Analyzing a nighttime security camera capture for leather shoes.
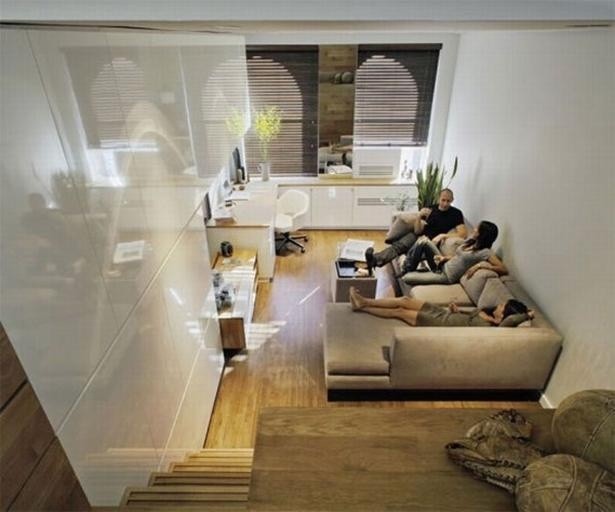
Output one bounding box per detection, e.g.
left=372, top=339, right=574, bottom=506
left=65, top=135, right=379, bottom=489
left=365, top=247, right=376, bottom=268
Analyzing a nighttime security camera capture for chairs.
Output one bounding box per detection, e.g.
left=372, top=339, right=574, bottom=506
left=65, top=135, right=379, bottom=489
left=275, top=189, right=310, bottom=255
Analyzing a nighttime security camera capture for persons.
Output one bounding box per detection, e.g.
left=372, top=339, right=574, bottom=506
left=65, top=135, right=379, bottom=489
left=21, top=193, right=66, bottom=238
left=366, top=189, right=508, bottom=285
left=349, top=286, right=535, bottom=327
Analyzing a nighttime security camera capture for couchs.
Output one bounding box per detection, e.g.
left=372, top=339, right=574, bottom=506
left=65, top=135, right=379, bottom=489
left=320, top=209, right=565, bottom=397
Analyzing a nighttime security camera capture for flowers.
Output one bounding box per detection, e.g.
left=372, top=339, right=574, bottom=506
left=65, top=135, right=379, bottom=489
left=253, top=104, right=283, bottom=162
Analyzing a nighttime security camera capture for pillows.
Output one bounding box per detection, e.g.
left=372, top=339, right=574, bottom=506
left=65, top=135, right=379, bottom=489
left=384, top=215, right=417, bottom=245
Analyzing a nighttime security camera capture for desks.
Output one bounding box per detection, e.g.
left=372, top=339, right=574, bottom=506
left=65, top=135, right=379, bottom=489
left=208, top=180, right=280, bottom=285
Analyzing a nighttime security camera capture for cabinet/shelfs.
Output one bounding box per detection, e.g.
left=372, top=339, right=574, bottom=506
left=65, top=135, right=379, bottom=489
left=210, top=248, right=261, bottom=353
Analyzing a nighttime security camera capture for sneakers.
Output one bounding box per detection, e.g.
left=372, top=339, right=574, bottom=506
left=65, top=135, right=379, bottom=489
left=350, top=286, right=369, bottom=312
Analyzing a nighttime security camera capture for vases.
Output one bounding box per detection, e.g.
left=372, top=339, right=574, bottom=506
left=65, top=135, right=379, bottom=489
left=259, top=163, right=270, bottom=181
